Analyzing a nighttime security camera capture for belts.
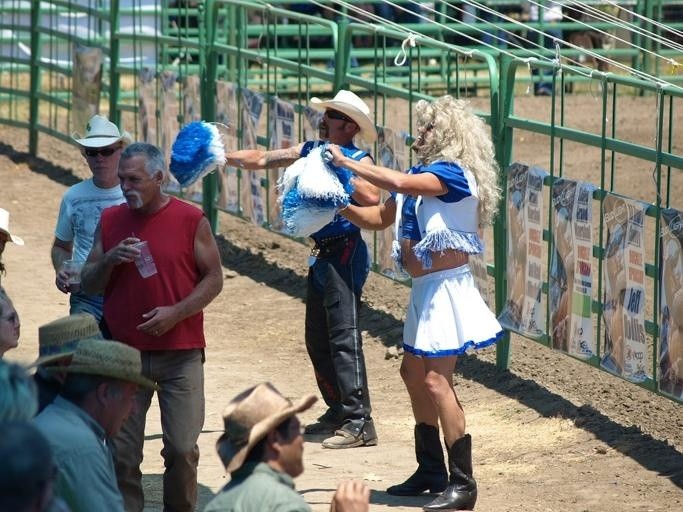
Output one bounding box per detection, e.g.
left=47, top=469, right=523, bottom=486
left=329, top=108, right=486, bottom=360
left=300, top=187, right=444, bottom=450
left=307, top=231, right=361, bottom=268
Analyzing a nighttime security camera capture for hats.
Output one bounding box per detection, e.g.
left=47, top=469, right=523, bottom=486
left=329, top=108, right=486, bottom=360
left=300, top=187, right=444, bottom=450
left=0, top=208, right=24, bottom=246
left=44, top=337, right=161, bottom=391
left=214, top=381, right=319, bottom=474
left=70, top=115, right=131, bottom=151
left=309, top=89, right=378, bottom=144
left=25, top=312, right=104, bottom=370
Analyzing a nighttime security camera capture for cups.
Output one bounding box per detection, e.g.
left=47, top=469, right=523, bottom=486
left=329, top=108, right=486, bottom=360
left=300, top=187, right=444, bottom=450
left=62, top=259, right=82, bottom=294
left=129, top=241, right=157, bottom=280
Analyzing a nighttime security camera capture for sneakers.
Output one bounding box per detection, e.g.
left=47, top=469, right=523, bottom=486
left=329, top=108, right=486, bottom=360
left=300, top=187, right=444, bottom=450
left=303, top=422, right=341, bottom=434
left=321, top=428, right=378, bottom=449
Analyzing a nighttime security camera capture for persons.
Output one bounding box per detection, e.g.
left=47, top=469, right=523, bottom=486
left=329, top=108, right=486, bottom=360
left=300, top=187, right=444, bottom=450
left=0, top=414, right=67, bottom=511
left=80, top=144, right=224, bottom=512
left=0, top=285, right=20, bottom=365
left=49, top=116, right=134, bottom=323
left=521, top=0, right=567, bottom=97
left=324, top=97, right=501, bottom=511
left=30, top=338, right=142, bottom=512
left=202, top=381, right=370, bottom=511
left=223, top=90, right=379, bottom=449
left=0, top=227, right=8, bottom=291
left=580, top=2, right=623, bottom=89
left=0, top=356, right=35, bottom=428
left=27, top=313, right=108, bottom=420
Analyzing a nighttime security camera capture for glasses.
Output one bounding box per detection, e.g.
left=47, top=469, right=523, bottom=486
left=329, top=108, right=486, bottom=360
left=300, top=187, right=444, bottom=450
left=83, top=146, right=121, bottom=157
left=323, top=110, right=352, bottom=123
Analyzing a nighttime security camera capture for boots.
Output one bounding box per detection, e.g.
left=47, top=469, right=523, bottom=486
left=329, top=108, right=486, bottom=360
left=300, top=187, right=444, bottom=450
left=385, top=422, right=448, bottom=496
left=422, top=433, right=477, bottom=511
